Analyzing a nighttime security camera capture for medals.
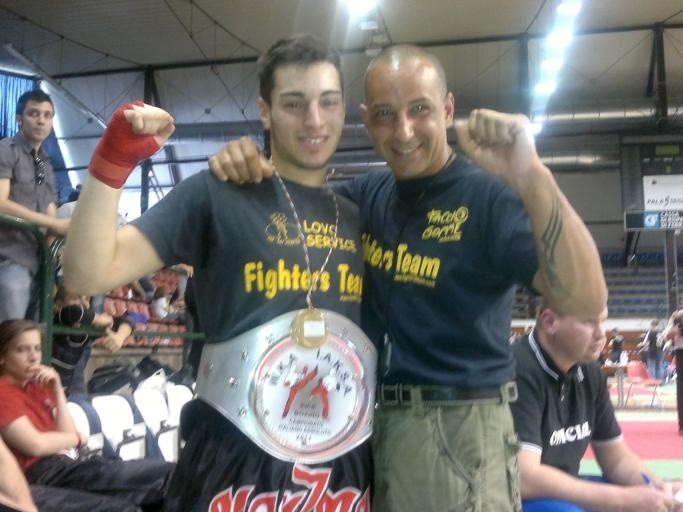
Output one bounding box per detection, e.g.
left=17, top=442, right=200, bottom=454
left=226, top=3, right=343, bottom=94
left=288, top=308, right=330, bottom=350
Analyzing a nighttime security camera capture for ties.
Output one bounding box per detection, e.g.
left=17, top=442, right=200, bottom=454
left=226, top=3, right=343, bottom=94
left=28, top=153, right=52, bottom=186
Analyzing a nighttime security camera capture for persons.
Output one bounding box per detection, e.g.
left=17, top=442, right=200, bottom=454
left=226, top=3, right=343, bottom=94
left=208, top=43, right=608, bottom=512
left=509, top=308, right=683, bottom=436
left=1, top=183, right=203, bottom=512
left=508, top=294, right=683, bottom=512
left=63, top=34, right=388, bottom=512
left=0, top=90, right=71, bottom=321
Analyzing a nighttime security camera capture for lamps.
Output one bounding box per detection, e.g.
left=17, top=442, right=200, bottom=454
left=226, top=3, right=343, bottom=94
left=365, top=43, right=383, bottom=56
left=360, top=14, right=379, bottom=30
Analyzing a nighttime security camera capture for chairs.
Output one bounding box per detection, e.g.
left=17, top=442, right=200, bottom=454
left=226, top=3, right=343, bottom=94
left=94, top=395, right=154, bottom=461
left=102, top=270, right=188, bottom=347
left=605, top=266, right=683, bottom=316
left=166, top=386, right=195, bottom=411
left=624, top=361, right=662, bottom=407
left=135, top=388, right=178, bottom=464
left=62, top=400, right=105, bottom=462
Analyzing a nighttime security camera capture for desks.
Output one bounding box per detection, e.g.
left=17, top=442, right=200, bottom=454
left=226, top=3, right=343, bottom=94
left=603, top=365, right=627, bottom=409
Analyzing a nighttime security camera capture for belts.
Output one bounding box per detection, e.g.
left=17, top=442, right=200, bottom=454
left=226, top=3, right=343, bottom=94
left=372, top=382, right=519, bottom=403
